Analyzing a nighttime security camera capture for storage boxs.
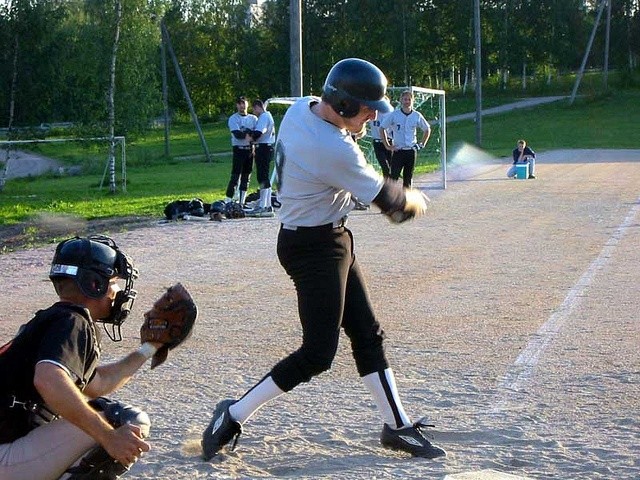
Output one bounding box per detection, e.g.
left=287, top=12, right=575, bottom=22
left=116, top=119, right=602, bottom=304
left=516, top=162, right=530, bottom=179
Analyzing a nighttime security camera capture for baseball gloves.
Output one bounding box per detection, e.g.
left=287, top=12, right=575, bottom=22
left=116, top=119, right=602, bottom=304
left=141, top=281, right=197, bottom=370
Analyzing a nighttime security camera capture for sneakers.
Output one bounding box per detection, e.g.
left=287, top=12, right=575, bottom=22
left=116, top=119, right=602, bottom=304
left=239, top=203, right=253, bottom=211
left=255, top=207, right=275, bottom=217
left=380, top=414, right=447, bottom=461
left=528, top=175, right=537, bottom=179
left=200, top=397, right=243, bottom=461
left=244, top=206, right=263, bottom=217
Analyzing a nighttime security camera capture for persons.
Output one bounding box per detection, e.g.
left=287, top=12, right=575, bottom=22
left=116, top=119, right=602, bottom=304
left=507, top=139, right=537, bottom=179
left=369, top=95, right=397, bottom=180
left=251, top=100, right=278, bottom=212
left=380, top=91, right=432, bottom=193
left=201, top=57, right=448, bottom=463
left=224, top=95, right=258, bottom=211
left=0, top=233, right=164, bottom=480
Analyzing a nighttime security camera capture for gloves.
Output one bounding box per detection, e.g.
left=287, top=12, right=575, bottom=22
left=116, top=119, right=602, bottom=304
left=413, top=142, right=424, bottom=152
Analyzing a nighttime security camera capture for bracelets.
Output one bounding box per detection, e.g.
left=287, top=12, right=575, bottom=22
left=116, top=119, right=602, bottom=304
left=135, top=338, right=160, bottom=363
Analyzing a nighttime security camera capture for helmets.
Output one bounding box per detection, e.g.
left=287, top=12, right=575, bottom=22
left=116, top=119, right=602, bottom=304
left=323, top=58, right=394, bottom=116
left=48, top=234, right=139, bottom=343
left=186, top=197, right=204, bottom=217
left=225, top=201, right=245, bottom=219
left=209, top=200, right=226, bottom=221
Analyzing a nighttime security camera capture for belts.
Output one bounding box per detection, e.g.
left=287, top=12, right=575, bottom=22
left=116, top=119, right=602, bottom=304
left=280, top=214, right=349, bottom=236
left=373, top=139, right=391, bottom=143
